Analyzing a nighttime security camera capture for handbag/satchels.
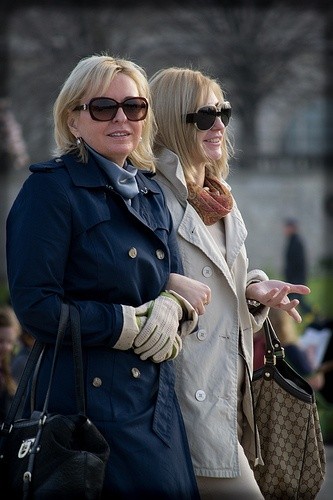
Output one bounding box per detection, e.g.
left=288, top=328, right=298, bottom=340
left=252, top=318, right=326, bottom=500
left=0, top=304, right=109, bottom=499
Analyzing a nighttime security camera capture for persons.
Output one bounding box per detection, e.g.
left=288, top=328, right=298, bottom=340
left=0, top=306, right=34, bottom=419
left=149, top=67, right=312, bottom=500
left=269, top=307, right=324, bottom=389
left=283, top=218, right=313, bottom=314
left=6, top=56, right=199, bottom=500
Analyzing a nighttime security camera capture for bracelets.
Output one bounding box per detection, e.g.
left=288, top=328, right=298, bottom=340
left=247, top=300, right=260, bottom=307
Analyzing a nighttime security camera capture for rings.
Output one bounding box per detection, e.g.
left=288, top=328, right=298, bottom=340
left=204, top=301, right=207, bottom=304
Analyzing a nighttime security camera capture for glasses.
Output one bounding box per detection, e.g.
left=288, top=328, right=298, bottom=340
left=71, top=96, right=148, bottom=123
left=179, top=101, right=231, bottom=131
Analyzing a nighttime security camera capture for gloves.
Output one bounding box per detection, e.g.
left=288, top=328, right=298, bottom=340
left=134, top=294, right=186, bottom=361
left=133, top=315, right=182, bottom=361
